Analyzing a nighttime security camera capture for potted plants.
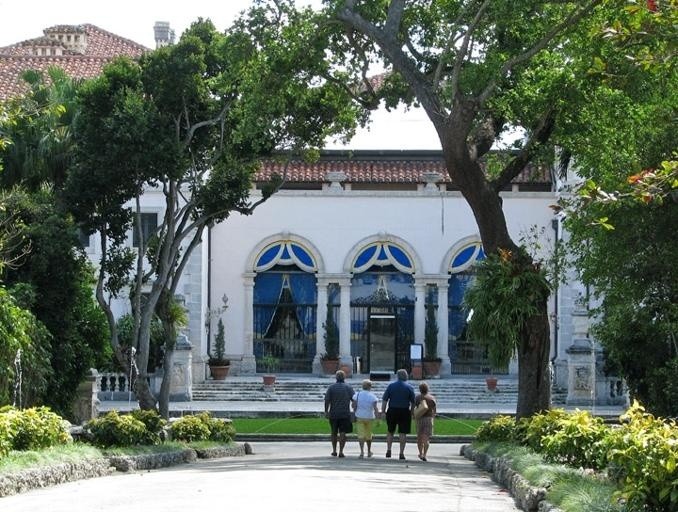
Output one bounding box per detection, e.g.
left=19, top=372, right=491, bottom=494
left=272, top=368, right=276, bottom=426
left=207, top=317, right=231, bottom=379
left=257, top=353, right=281, bottom=386
left=421, top=285, right=442, bottom=377
left=319, top=303, right=341, bottom=374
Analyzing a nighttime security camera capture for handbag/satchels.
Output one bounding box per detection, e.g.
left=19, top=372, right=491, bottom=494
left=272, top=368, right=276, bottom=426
left=350, top=412, right=356, bottom=423
left=414, top=399, right=429, bottom=420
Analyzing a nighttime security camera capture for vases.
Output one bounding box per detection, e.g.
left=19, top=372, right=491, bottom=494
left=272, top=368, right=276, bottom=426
left=486, top=378, right=498, bottom=390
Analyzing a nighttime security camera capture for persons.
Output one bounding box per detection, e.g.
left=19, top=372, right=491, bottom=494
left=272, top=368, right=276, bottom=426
left=381, top=367, right=415, bottom=458
left=323, top=370, right=355, bottom=457
left=410, top=382, right=437, bottom=462
left=352, top=378, right=380, bottom=457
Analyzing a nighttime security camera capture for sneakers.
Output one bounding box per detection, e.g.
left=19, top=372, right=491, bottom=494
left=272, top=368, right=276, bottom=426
left=332, top=452, right=337, bottom=456
left=400, top=454, right=405, bottom=459
left=360, top=451, right=374, bottom=457
left=339, top=453, right=344, bottom=457
left=418, top=454, right=427, bottom=461
left=386, top=452, right=391, bottom=458
